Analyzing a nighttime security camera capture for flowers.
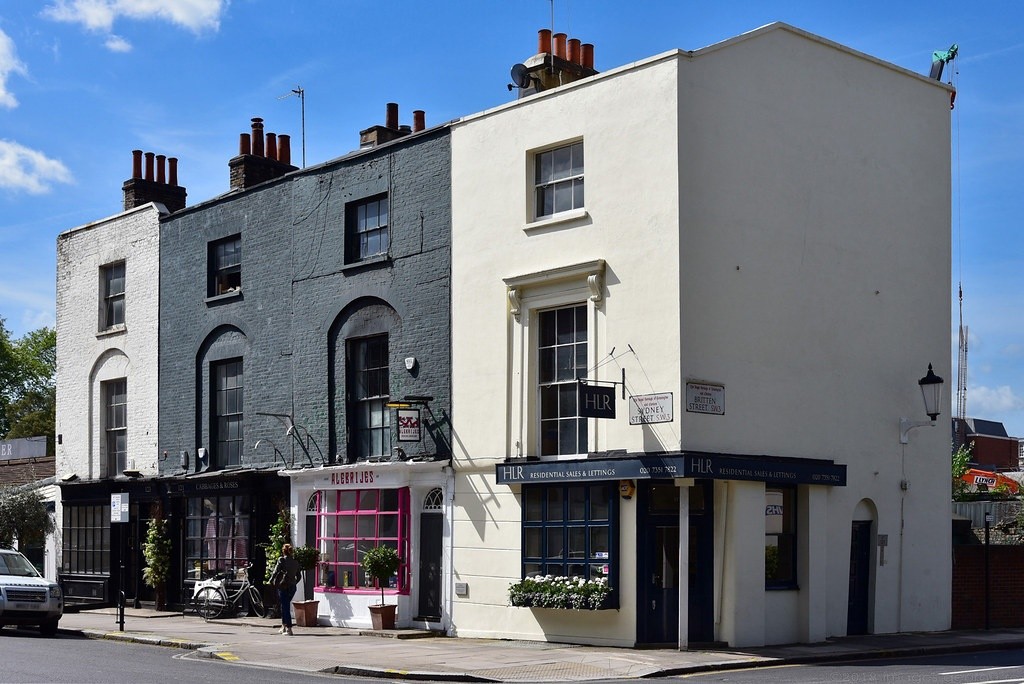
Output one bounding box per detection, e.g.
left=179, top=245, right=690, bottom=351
left=507, top=575, right=612, bottom=611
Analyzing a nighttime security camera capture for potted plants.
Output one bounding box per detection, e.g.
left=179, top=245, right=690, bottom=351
left=292, top=544, right=320, bottom=627
left=362, top=545, right=400, bottom=630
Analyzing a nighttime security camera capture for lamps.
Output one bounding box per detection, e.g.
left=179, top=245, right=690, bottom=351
left=899, top=363, right=944, bottom=445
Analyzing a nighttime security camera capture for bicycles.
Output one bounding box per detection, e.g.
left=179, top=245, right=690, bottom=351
left=194, top=563, right=266, bottom=619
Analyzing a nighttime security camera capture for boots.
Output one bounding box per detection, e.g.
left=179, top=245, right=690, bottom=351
left=282, top=626, right=293, bottom=636
left=278, top=623, right=287, bottom=634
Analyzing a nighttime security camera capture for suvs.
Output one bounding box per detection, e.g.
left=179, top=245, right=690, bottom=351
left=0, top=542, right=64, bottom=638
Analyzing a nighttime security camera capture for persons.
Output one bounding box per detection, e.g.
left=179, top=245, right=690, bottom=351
left=268, top=544, right=301, bottom=635
left=203, top=497, right=247, bottom=570
